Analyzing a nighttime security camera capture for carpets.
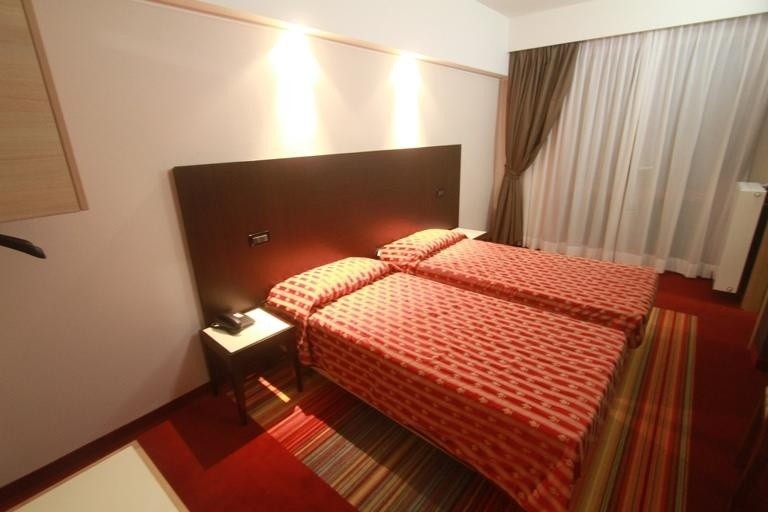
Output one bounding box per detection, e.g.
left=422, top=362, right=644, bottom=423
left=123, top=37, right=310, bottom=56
left=224, top=305, right=699, bottom=512
left=4, top=440, right=191, bottom=512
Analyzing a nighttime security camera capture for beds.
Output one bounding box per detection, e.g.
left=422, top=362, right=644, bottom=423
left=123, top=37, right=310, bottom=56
left=264, top=254, right=630, bottom=511
left=377, top=229, right=659, bottom=347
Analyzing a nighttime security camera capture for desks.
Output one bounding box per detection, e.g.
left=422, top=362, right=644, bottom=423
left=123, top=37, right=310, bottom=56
left=199, top=305, right=303, bottom=426
left=451, top=227, right=489, bottom=243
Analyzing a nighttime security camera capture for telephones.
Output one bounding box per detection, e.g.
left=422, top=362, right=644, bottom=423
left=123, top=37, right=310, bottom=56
left=208, top=310, right=256, bottom=335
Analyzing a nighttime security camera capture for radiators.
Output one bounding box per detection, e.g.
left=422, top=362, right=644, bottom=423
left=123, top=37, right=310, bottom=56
left=709, top=181, right=768, bottom=300
